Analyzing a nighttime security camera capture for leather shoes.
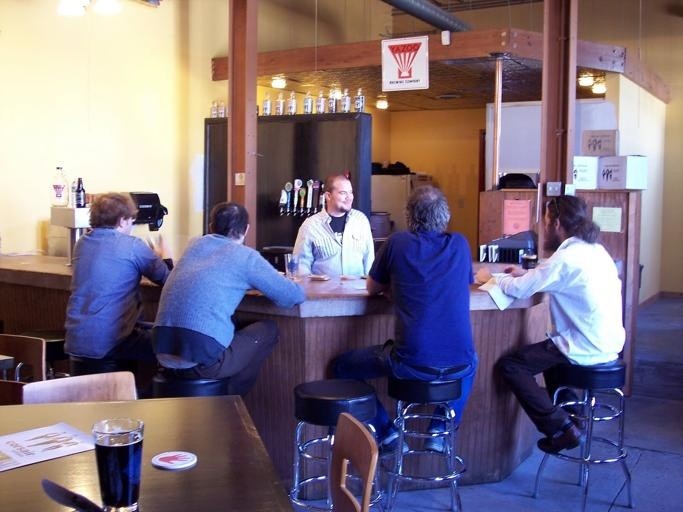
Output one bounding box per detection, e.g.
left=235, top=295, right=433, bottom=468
left=568, top=413, right=586, bottom=429
left=537, top=426, right=586, bottom=454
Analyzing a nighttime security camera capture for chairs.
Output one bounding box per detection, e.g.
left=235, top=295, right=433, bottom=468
left=331, top=413, right=379, bottom=511
left=23, top=371, right=138, bottom=405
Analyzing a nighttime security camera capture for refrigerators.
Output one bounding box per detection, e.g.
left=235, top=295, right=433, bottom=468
left=371, top=174, right=431, bottom=234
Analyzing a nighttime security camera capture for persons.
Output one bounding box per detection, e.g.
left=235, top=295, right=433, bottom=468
left=149, top=201, right=306, bottom=400
left=64, top=192, right=172, bottom=377
left=326, top=183, right=479, bottom=461
left=291, top=173, right=376, bottom=282
left=476, top=194, right=627, bottom=454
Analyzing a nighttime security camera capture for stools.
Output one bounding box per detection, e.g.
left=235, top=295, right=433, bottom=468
left=148, top=378, right=229, bottom=397
left=52, top=360, right=128, bottom=381
left=532, top=360, right=634, bottom=511
left=388, top=378, right=470, bottom=511
left=287, top=379, right=388, bottom=511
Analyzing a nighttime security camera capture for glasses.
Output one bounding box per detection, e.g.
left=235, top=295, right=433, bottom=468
left=550, top=195, right=566, bottom=214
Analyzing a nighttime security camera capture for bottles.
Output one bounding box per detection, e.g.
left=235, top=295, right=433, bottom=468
left=218, top=101, right=225, bottom=117
left=328, top=89, right=338, bottom=113
left=354, top=89, right=364, bottom=112
left=70, top=181, right=77, bottom=206
left=303, top=93, right=312, bottom=114
left=522, top=248, right=537, bottom=268
left=210, top=102, right=216, bottom=118
left=315, top=90, right=325, bottom=113
left=76, top=178, right=86, bottom=207
left=48, top=168, right=69, bottom=207
left=341, top=88, right=351, bottom=113
left=263, top=93, right=271, bottom=115
left=288, top=92, right=297, bottom=115
left=275, top=93, right=284, bottom=115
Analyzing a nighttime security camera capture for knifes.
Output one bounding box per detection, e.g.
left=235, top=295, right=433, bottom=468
left=41, top=478, right=104, bottom=512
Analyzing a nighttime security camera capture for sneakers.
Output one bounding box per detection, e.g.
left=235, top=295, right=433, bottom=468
left=424, top=434, right=450, bottom=454
left=375, top=435, right=410, bottom=460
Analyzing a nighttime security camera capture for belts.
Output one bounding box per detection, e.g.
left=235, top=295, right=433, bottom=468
left=155, top=359, right=209, bottom=377
left=389, top=350, right=469, bottom=375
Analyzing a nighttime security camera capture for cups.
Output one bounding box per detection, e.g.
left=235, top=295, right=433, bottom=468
left=92, top=417, right=144, bottom=511
left=286, top=255, right=299, bottom=281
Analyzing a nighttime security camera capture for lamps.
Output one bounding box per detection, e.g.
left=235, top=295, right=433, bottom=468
left=376, top=95, right=389, bottom=108
